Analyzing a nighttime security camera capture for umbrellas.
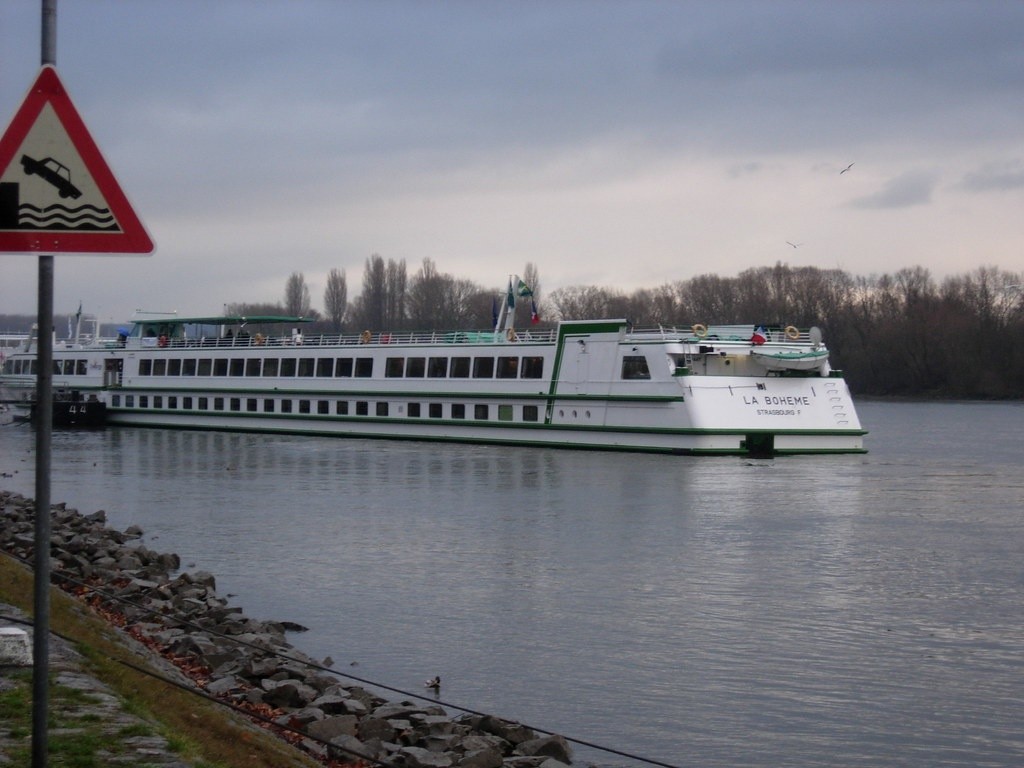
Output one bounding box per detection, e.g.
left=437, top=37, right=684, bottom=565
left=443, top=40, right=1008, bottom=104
left=116, top=327, right=130, bottom=337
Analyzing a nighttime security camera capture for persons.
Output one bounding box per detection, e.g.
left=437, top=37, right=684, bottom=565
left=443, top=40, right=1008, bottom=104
left=764, top=317, right=783, bottom=342
left=225, top=329, right=250, bottom=346
left=294, top=328, right=303, bottom=346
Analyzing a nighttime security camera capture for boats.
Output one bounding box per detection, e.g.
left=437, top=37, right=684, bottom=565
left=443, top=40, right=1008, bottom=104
left=0, top=272, right=867, bottom=458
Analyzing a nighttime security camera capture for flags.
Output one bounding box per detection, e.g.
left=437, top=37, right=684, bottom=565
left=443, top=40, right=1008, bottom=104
left=753, top=323, right=768, bottom=345
left=492, top=279, right=539, bottom=331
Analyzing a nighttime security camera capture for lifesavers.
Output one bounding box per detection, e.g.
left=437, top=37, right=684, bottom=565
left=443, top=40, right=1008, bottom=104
left=160, top=336, right=165, bottom=346
left=362, top=330, right=371, bottom=343
left=256, top=334, right=262, bottom=343
left=785, top=326, right=799, bottom=340
left=506, top=328, right=514, bottom=341
left=693, top=324, right=707, bottom=337
